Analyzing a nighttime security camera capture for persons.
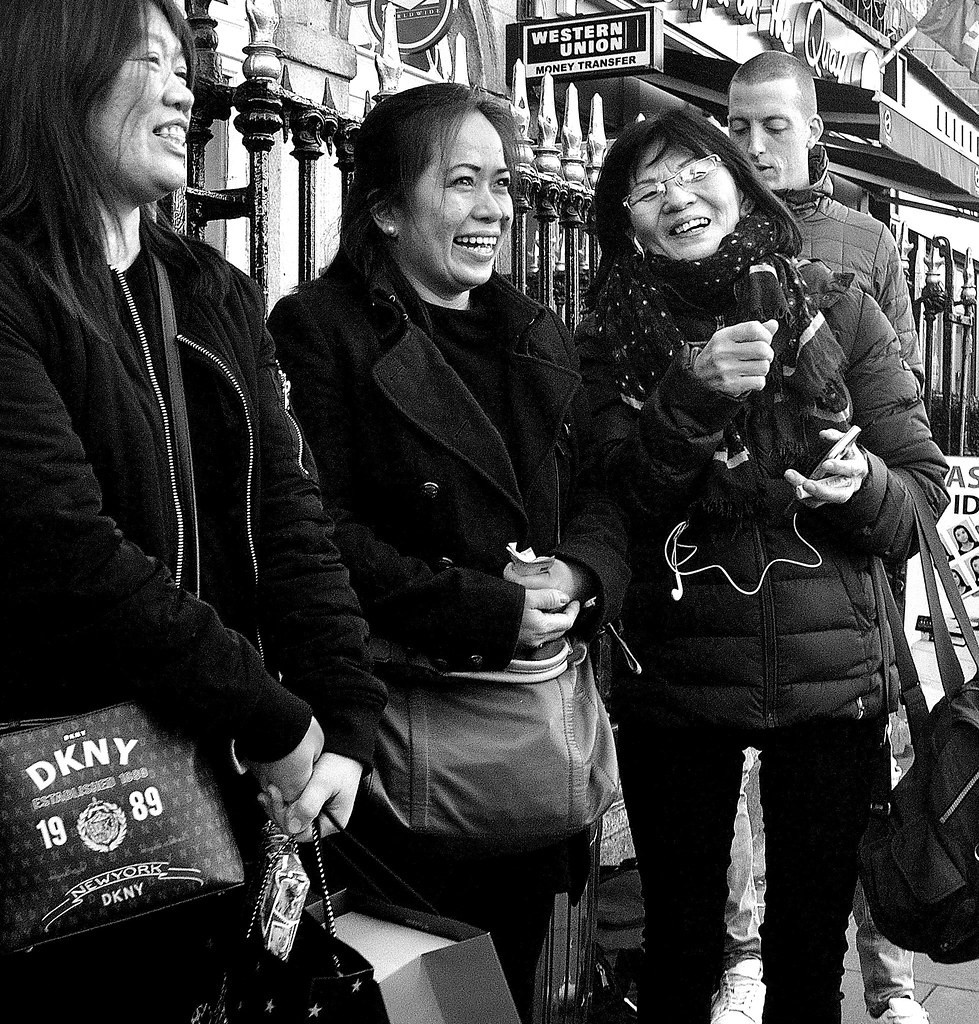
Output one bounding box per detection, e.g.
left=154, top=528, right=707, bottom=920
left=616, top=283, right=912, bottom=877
left=270, top=83, right=634, bottom=1024
left=572, top=108, right=950, bottom=1024
left=714, top=49, right=930, bottom=1024
left=0, top=0, right=385, bottom=1023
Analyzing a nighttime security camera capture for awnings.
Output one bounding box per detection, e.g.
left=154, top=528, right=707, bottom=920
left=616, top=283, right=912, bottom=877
left=634, top=48, right=979, bottom=203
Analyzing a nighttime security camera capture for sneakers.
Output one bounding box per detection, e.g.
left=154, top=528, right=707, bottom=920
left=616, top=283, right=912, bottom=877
left=709, top=959, right=768, bottom=1024
left=866, top=998, right=931, bottom=1024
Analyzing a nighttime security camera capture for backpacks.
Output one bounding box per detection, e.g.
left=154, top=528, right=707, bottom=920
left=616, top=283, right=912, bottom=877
left=853, top=467, right=978, bottom=962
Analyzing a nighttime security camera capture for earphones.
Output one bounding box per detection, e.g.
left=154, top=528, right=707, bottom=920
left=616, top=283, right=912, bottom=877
left=671, top=571, right=685, bottom=602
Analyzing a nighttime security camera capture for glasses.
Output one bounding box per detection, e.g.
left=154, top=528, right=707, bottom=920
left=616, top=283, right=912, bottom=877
left=623, top=153, right=723, bottom=216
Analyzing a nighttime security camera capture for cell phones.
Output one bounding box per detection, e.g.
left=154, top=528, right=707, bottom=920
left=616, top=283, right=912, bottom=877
left=785, top=426, right=862, bottom=518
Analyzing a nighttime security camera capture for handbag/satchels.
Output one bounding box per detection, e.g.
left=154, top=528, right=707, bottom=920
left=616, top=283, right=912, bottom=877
left=298, top=810, right=523, bottom=1022
left=1, top=695, right=246, bottom=955
left=354, top=624, right=619, bottom=848
